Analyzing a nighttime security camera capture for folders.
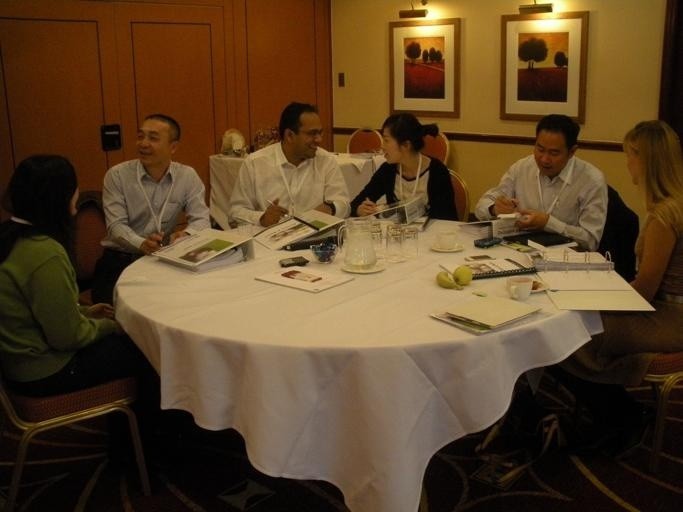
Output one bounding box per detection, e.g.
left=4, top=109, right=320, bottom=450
left=458, top=219, right=531, bottom=241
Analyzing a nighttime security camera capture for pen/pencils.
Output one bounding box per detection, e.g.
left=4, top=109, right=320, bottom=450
left=267, top=200, right=288, bottom=218
left=156, top=230, right=163, bottom=247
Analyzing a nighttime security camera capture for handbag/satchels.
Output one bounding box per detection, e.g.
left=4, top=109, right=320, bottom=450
left=515, top=369, right=585, bottom=467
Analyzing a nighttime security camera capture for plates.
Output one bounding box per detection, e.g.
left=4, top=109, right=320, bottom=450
left=531, top=280, right=549, bottom=294
left=431, top=245, right=465, bottom=253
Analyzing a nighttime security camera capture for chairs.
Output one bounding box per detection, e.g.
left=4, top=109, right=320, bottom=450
left=447, top=169, right=470, bottom=223
left=419, top=132, right=450, bottom=166
left=642, top=350, right=682, bottom=473
left=74, top=190, right=107, bottom=306
left=0, top=374, right=152, bottom=512
left=347, top=127, right=384, bottom=154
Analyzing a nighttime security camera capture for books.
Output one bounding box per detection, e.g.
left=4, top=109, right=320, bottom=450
left=439, top=255, right=537, bottom=277
left=253, top=265, right=354, bottom=294
left=457, top=211, right=533, bottom=239
left=433, top=297, right=541, bottom=333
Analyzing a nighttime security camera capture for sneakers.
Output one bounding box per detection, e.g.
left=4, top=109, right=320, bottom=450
left=607, top=405, right=655, bottom=459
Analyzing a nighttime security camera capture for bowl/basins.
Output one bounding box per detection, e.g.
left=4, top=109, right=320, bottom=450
left=310, top=244, right=337, bottom=263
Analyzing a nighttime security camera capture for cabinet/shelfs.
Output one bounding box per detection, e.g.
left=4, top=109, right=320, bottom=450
left=243, top=0, right=319, bottom=152
left=1, top=1, right=229, bottom=227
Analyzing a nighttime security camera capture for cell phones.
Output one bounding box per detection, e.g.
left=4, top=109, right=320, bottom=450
left=474, top=237, right=500, bottom=248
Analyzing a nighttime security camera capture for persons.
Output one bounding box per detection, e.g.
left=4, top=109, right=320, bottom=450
left=0, top=154, right=158, bottom=493
left=473, top=113, right=608, bottom=252
left=546, top=121, right=683, bottom=456
left=89, top=113, right=211, bottom=302
left=349, top=112, right=458, bottom=220
left=228, top=101, right=352, bottom=227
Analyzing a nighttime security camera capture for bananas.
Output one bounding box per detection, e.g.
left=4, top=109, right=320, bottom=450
left=437, top=272, right=463, bottom=289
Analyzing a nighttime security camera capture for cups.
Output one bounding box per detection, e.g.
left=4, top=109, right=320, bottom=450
left=373, top=224, right=419, bottom=261
left=433, top=231, right=457, bottom=250
left=506, top=276, right=533, bottom=301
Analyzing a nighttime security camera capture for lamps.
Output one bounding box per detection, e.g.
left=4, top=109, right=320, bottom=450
left=520, top=0, right=553, bottom=15
left=399, top=0, right=428, bottom=17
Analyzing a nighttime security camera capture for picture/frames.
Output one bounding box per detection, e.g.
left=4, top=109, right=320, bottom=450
left=500, top=11, right=589, bottom=123
left=388, top=18, right=460, bottom=120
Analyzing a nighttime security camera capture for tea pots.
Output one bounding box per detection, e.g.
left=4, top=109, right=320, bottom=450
left=338, top=217, right=377, bottom=270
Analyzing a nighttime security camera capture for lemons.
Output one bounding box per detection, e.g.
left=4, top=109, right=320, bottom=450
left=454, top=266, right=472, bottom=285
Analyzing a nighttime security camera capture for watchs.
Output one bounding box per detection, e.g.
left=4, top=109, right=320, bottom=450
left=320, top=199, right=336, bottom=216
